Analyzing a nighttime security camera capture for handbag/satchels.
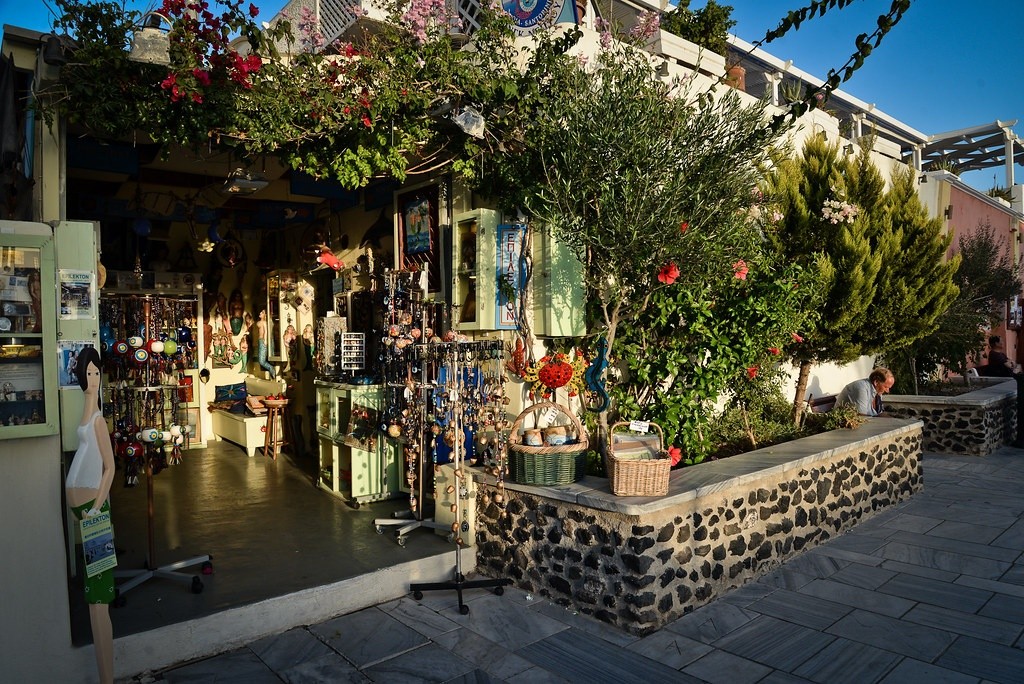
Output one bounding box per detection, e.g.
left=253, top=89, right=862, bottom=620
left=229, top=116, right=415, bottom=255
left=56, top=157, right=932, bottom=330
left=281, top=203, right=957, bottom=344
left=343, top=394, right=383, bottom=453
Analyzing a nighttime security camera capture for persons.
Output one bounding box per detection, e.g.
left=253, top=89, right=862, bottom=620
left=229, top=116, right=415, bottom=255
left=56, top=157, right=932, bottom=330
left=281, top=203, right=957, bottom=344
left=65, top=346, right=115, bottom=684
left=834, top=366, right=895, bottom=418
left=28, top=270, right=41, bottom=333
left=214, top=289, right=315, bottom=380
left=989, top=337, right=1016, bottom=377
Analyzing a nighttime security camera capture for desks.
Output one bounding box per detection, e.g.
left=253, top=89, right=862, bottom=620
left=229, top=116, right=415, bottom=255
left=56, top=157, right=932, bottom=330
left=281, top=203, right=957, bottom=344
left=212, top=409, right=284, bottom=457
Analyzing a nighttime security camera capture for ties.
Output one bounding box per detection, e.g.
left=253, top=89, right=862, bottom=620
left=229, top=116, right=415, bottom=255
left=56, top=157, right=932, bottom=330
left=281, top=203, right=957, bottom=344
left=875, top=393, right=882, bottom=414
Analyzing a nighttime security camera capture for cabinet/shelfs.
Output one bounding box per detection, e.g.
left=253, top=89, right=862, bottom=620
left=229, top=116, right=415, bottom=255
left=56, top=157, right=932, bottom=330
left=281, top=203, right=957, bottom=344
left=0, top=234, right=59, bottom=440
left=313, top=380, right=399, bottom=506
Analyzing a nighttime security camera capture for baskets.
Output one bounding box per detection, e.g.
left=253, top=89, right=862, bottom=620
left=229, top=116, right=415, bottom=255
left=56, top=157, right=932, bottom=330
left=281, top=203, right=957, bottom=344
left=606, top=422, right=672, bottom=497
left=507, top=402, right=589, bottom=486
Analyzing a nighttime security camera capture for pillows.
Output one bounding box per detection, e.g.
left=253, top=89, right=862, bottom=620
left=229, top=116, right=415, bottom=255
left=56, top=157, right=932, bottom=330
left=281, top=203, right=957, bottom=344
left=214, top=382, right=248, bottom=402
